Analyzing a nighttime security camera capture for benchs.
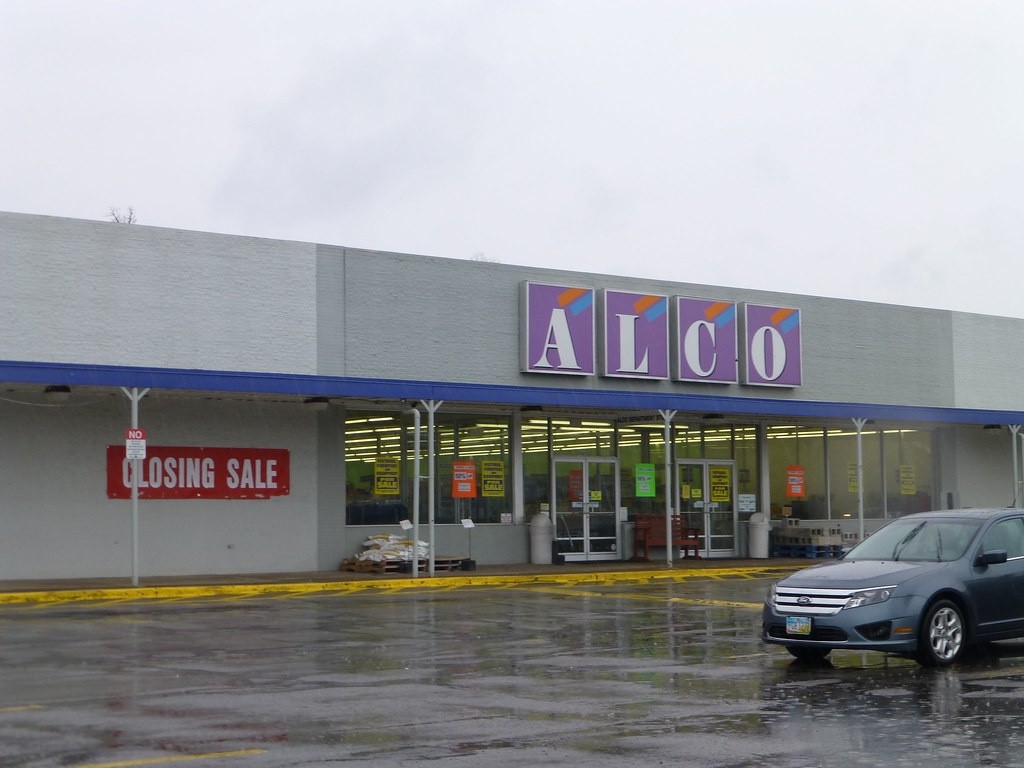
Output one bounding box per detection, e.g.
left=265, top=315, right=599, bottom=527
left=631, top=514, right=700, bottom=561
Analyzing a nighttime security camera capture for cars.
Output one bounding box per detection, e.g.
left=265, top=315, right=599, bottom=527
left=761, top=508, right=1024, bottom=665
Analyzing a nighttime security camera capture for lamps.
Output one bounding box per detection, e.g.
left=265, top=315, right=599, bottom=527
left=43, top=386, right=72, bottom=403
left=982, top=425, right=1002, bottom=435
left=303, top=397, right=330, bottom=412
left=701, top=414, right=724, bottom=425
left=520, top=405, right=545, bottom=417
left=863, top=421, right=876, bottom=428
